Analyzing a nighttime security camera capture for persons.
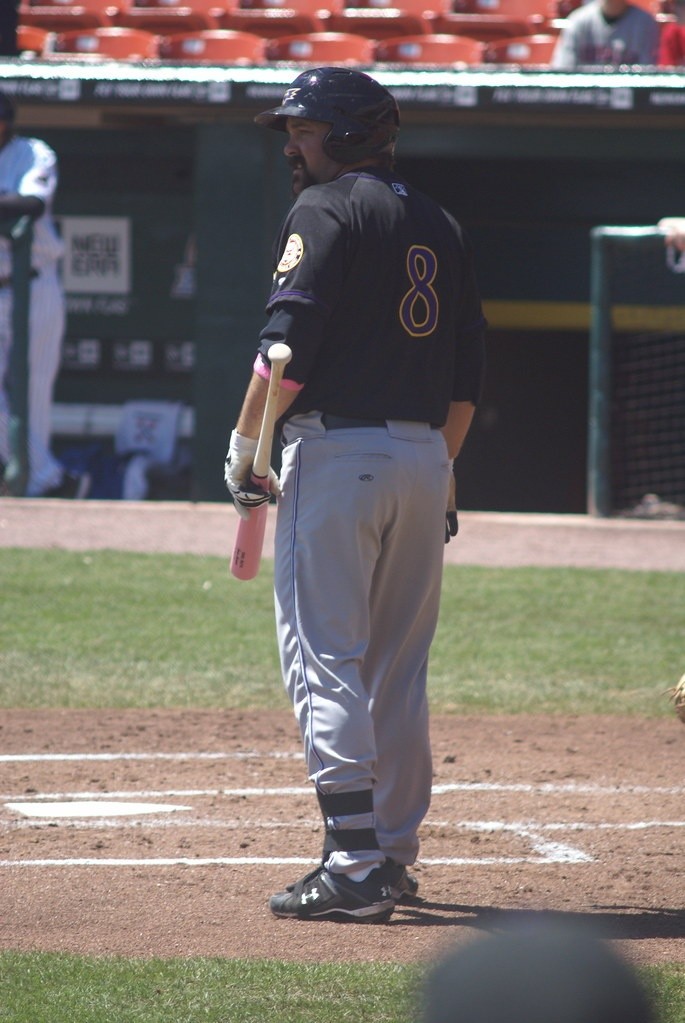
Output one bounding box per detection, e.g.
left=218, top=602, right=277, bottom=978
left=550, top=0, right=685, bottom=67
left=0, top=88, right=69, bottom=498
left=222, top=67, right=501, bottom=926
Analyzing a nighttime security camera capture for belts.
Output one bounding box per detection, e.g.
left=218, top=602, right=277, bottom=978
left=280, top=411, right=440, bottom=448
left=0, top=269, right=39, bottom=291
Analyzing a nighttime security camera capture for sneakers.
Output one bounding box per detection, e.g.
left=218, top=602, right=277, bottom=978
left=268, top=857, right=396, bottom=924
left=380, top=855, right=419, bottom=901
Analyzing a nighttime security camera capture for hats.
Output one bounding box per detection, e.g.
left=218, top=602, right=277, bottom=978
left=0, top=92, right=16, bottom=119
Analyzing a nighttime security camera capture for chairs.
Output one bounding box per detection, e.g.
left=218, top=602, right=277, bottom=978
left=15, top=0, right=665, bottom=65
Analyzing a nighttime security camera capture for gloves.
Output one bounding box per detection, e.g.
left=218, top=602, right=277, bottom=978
left=444, top=459, right=458, bottom=543
left=223, top=428, right=282, bottom=522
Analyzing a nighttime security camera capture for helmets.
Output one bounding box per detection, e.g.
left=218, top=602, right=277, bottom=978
left=253, top=68, right=400, bottom=164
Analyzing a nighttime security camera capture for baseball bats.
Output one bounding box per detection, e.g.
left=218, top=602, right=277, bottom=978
left=229, top=340, right=294, bottom=582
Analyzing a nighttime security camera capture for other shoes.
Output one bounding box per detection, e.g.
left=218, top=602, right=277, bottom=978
left=629, top=501, right=682, bottom=520
left=5, top=461, right=84, bottom=501
